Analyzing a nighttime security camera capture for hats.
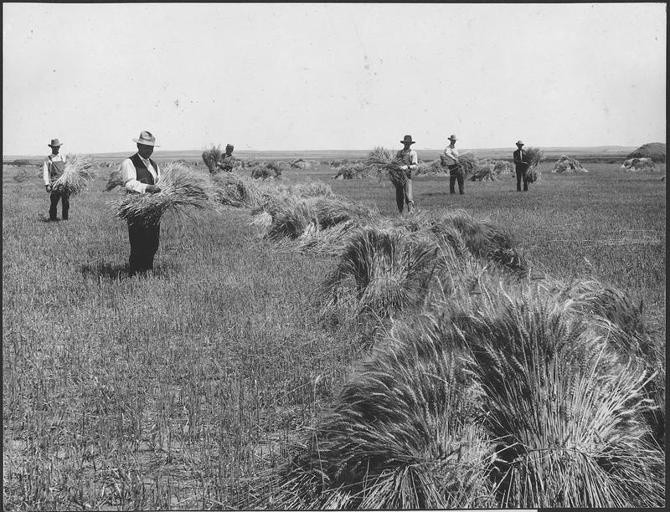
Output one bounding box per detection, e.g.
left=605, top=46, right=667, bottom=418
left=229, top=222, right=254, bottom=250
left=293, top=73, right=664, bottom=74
left=132, top=130, right=163, bottom=148
left=400, top=135, right=416, bottom=144
left=515, top=140, right=525, bottom=145
left=447, top=134, right=459, bottom=142
left=47, top=138, right=64, bottom=148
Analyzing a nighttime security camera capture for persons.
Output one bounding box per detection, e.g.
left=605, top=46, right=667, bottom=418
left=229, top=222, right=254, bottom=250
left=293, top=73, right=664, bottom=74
left=119, top=130, right=162, bottom=273
left=512, top=140, right=529, bottom=192
left=444, top=134, right=466, bottom=194
left=394, top=134, right=418, bottom=214
left=218, top=144, right=235, bottom=172
left=43, top=137, right=71, bottom=221
